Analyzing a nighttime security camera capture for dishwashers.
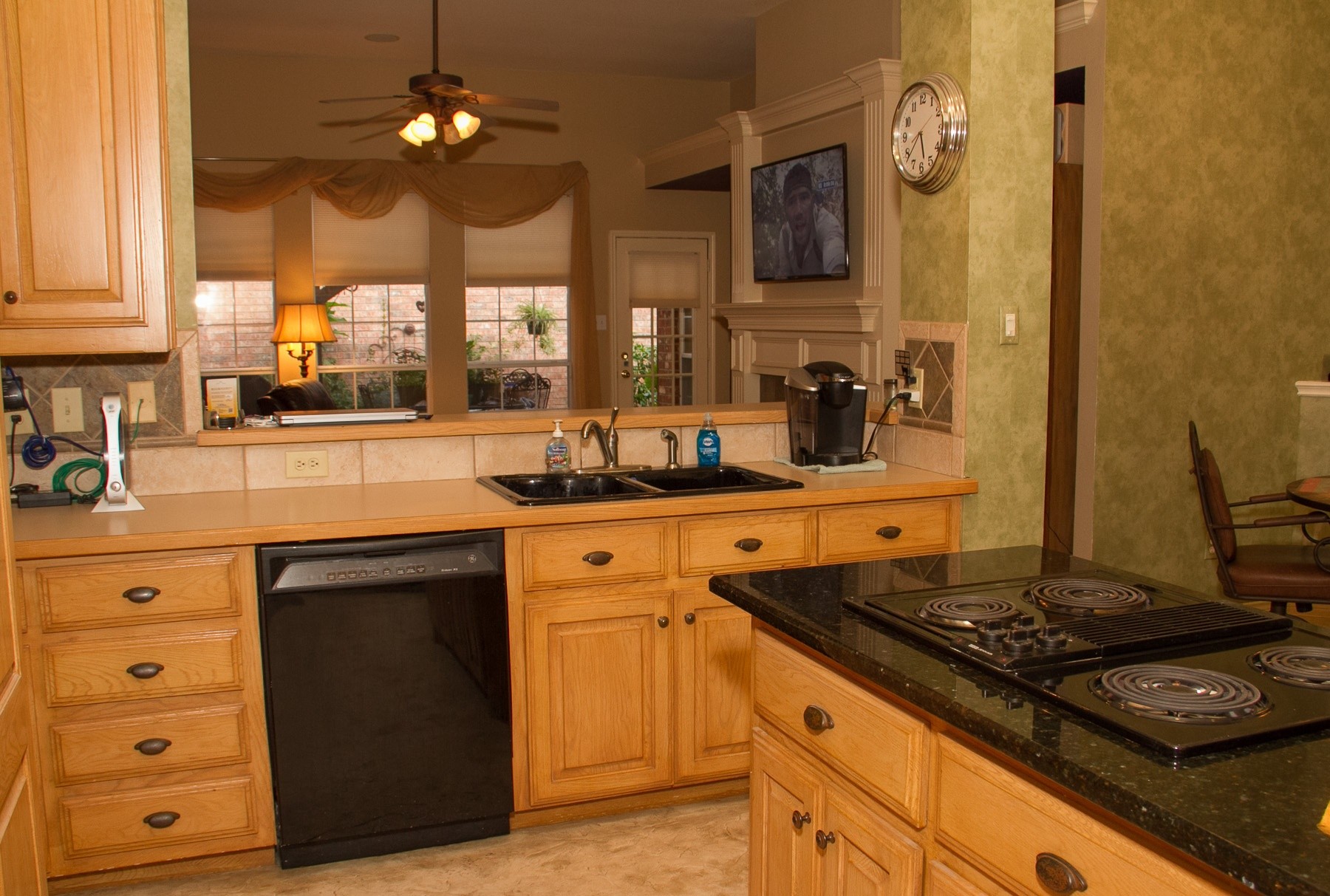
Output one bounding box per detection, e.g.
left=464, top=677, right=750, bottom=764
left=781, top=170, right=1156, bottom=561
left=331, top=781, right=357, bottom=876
left=255, top=529, right=515, bottom=869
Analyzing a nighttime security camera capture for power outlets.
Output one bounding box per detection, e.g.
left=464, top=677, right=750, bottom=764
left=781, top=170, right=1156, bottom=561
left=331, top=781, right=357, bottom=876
left=285, top=448, right=327, bottom=480
left=2, top=389, right=34, bottom=437
left=904, top=368, right=923, bottom=409
left=51, top=385, right=84, bottom=433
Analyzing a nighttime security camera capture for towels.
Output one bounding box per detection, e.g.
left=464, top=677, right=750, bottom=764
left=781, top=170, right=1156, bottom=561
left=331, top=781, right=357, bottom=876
left=773, top=455, right=888, bottom=475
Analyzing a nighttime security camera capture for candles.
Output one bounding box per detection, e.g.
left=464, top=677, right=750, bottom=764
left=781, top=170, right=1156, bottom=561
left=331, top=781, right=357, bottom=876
left=510, top=302, right=560, bottom=357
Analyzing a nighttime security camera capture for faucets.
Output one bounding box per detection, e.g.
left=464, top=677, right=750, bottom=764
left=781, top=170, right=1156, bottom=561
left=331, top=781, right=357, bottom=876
left=580, top=405, right=620, bottom=473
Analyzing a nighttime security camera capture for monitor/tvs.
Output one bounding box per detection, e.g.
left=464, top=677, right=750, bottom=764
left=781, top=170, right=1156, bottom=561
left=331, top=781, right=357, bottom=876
left=750, top=144, right=851, bottom=284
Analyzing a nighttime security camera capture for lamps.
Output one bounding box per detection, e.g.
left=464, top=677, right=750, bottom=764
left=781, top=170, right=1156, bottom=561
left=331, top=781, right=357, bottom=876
left=268, top=302, right=341, bottom=380
left=397, top=100, right=481, bottom=148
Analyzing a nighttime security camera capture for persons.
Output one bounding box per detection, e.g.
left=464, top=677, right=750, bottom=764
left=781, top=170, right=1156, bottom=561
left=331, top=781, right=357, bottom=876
left=778, top=162, right=851, bottom=281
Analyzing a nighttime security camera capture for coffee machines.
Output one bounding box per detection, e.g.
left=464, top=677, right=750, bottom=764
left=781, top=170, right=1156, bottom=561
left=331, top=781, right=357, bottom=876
left=783, top=360, right=870, bottom=466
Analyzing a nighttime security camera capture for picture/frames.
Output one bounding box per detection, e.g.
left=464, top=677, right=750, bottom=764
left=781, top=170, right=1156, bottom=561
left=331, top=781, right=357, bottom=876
left=748, top=142, right=852, bottom=284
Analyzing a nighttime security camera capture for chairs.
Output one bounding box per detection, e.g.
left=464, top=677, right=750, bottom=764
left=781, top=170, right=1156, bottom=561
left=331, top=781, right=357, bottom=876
left=1180, top=420, right=1326, bottom=625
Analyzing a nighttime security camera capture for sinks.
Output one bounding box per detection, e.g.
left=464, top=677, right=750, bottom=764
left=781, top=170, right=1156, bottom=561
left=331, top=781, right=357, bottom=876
left=622, top=459, right=805, bottom=499
left=475, top=467, right=661, bottom=506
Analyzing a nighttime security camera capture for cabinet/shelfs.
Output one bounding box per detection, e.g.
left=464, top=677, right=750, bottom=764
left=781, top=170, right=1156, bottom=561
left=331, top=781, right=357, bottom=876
left=737, top=606, right=1272, bottom=896
left=11, top=492, right=274, bottom=877
left=504, top=446, right=980, bottom=834
left=2, top=0, right=184, bottom=362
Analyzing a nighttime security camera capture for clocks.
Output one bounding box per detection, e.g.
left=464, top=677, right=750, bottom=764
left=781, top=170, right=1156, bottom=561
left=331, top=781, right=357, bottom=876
left=890, top=74, right=971, bottom=196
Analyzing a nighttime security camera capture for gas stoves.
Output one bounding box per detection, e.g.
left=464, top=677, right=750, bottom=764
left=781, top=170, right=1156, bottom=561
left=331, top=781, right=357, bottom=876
left=841, top=566, right=1330, bottom=761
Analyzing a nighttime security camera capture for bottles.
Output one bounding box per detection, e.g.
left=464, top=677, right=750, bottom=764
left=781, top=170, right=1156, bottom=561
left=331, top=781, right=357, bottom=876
left=698, top=412, right=721, bottom=468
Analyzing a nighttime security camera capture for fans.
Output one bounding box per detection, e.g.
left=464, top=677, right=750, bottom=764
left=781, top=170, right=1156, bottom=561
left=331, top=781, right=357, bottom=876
left=319, top=2, right=563, bottom=130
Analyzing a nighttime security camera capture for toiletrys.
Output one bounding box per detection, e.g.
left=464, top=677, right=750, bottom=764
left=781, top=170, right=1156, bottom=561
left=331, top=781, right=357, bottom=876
left=696, top=412, right=722, bottom=467
left=542, top=419, right=573, bottom=475
